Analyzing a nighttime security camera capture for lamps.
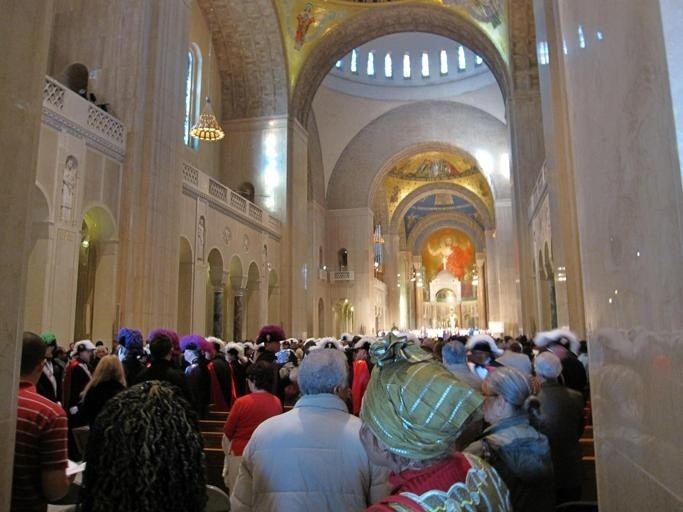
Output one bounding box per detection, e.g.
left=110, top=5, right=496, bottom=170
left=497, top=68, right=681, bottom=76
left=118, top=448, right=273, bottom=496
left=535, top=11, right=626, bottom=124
left=187, top=2, right=237, bottom=143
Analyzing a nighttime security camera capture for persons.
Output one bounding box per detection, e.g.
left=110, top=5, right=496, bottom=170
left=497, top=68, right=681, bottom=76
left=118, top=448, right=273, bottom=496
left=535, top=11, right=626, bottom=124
left=60, top=158, right=75, bottom=226
left=197, top=217, right=203, bottom=260
left=224, top=327, right=353, bottom=489
left=9, top=327, right=255, bottom=512
left=228, top=348, right=395, bottom=511
left=426, top=235, right=472, bottom=278
left=340, top=326, right=589, bottom=512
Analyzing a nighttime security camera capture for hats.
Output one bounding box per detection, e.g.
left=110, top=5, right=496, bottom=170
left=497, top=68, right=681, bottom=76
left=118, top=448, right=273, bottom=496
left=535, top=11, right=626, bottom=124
left=466, top=334, right=505, bottom=355
left=42, top=326, right=421, bottom=356
left=533, top=327, right=578, bottom=355
